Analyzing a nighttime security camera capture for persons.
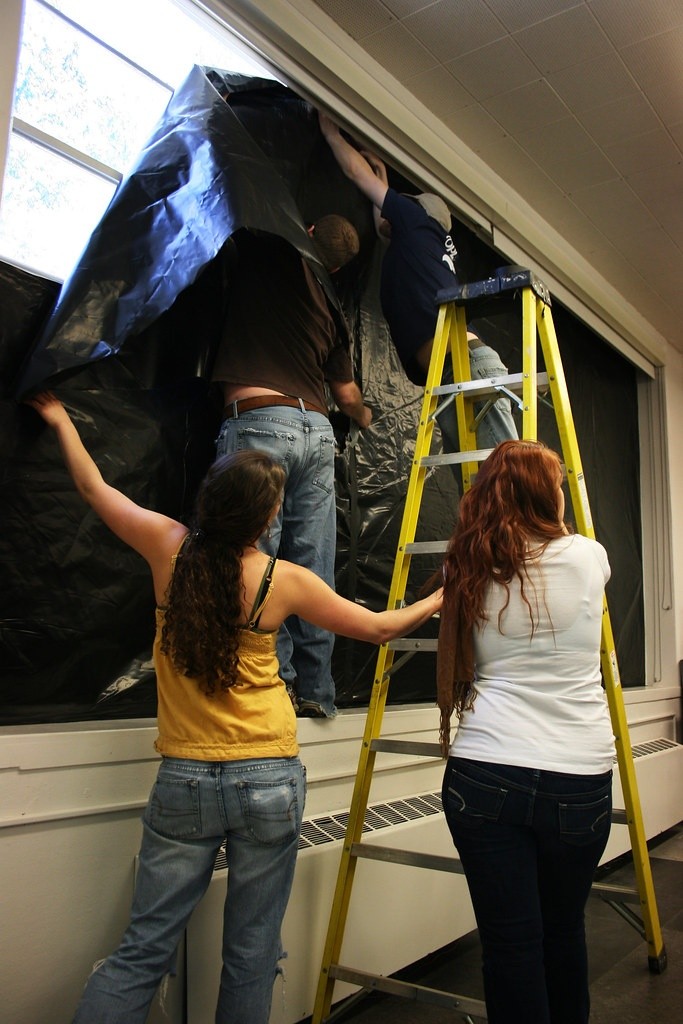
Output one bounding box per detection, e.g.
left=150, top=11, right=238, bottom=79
left=30, top=385, right=445, bottom=1024
left=437, top=439, right=613, bottom=1023
left=317, top=109, right=520, bottom=499
left=82, top=214, right=375, bottom=718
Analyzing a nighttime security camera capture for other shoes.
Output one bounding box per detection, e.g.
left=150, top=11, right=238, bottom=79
left=289, top=692, right=299, bottom=712
left=297, top=703, right=324, bottom=717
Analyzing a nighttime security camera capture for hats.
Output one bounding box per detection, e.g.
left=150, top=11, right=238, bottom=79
left=400, top=193, right=452, bottom=234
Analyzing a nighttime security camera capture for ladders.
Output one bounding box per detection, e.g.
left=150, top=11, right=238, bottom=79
left=301, top=268, right=671, bottom=1024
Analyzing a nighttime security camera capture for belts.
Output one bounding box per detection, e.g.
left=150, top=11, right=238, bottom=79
left=221, top=396, right=328, bottom=421
